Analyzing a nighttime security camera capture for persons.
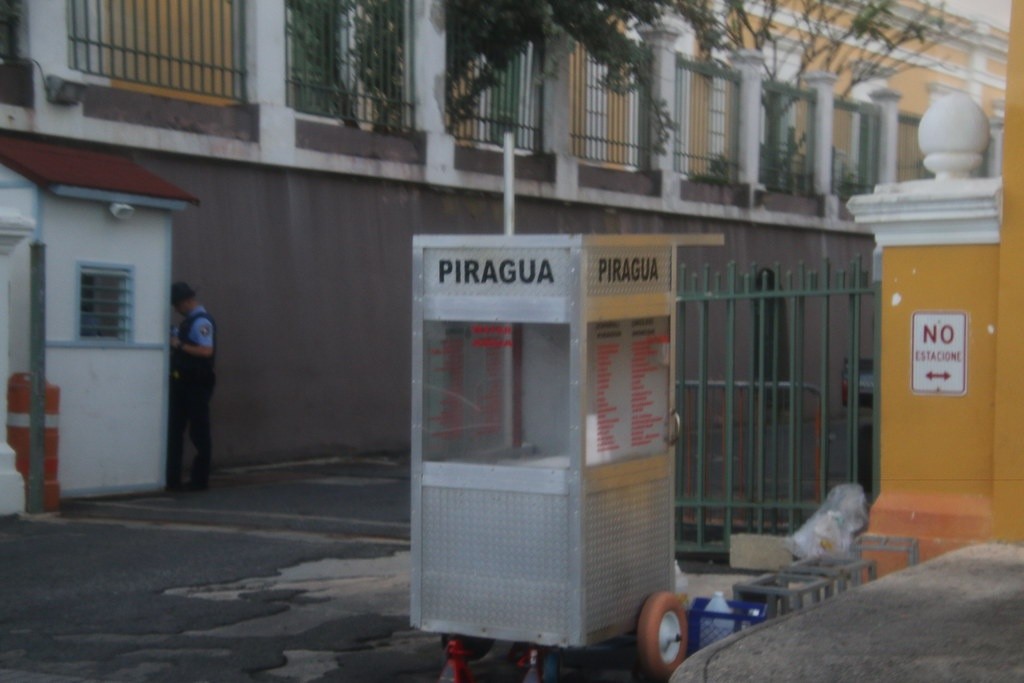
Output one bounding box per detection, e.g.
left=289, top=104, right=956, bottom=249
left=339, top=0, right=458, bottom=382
left=167, top=282, right=216, bottom=492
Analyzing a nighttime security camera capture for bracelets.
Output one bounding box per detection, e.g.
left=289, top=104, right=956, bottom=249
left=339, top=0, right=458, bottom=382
left=176, top=341, right=184, bottom=350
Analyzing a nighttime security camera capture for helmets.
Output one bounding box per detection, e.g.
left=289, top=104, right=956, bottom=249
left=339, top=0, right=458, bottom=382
left=171, top=281, right=196, bottom=306
